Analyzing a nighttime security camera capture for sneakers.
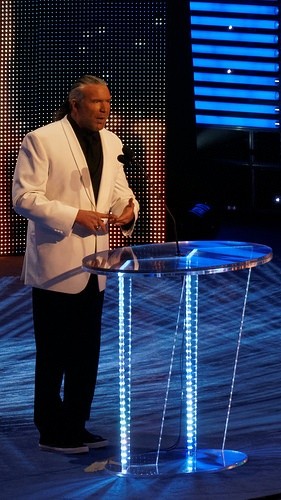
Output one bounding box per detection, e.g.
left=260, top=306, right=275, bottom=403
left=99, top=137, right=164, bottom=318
left=39, top=438, right=88, bottom=454
left=82, top=431, right=110, bottom=450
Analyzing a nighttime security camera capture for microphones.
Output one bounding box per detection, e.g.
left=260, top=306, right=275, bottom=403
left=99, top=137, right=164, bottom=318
left=122, top=145, right=186, bottom=256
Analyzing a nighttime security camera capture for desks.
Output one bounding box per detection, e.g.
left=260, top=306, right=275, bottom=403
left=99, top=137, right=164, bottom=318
left=83, top=240, right=274, bottom=478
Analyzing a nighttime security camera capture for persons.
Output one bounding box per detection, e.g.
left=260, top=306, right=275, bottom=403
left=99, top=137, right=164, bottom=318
left=12, top=74, right=139, bottom=454
